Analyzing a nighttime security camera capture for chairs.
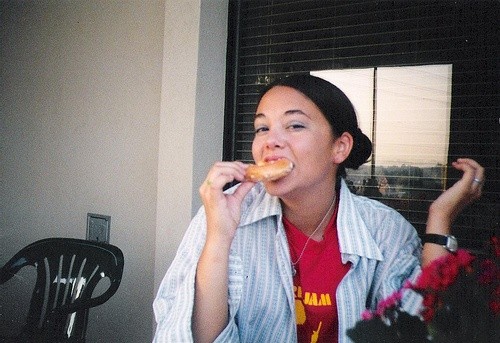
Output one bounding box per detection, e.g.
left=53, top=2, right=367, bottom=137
left=0, top=238, right=123, bottom=343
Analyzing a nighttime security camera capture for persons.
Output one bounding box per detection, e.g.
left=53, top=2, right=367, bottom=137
left=151, top=73, right=486, bottom=342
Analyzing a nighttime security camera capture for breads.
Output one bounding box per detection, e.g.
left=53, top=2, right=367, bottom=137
left=243, top=158, right=293, bottom=183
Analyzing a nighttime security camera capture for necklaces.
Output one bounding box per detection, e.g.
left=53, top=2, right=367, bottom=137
left=283, top=191, right=337, bottom=277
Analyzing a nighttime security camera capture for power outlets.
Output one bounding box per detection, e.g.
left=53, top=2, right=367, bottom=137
left=88, top=212, right=109, bottom=243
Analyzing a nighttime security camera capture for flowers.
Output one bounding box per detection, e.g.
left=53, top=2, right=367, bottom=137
left=345, top=236, right=500, bottom=343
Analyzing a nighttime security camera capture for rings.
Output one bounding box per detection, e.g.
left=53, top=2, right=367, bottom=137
left=474, top=178, right=482, bottom=185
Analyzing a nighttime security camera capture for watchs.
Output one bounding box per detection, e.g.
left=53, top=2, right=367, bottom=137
left=422, top=233, right=458, bottom=253
